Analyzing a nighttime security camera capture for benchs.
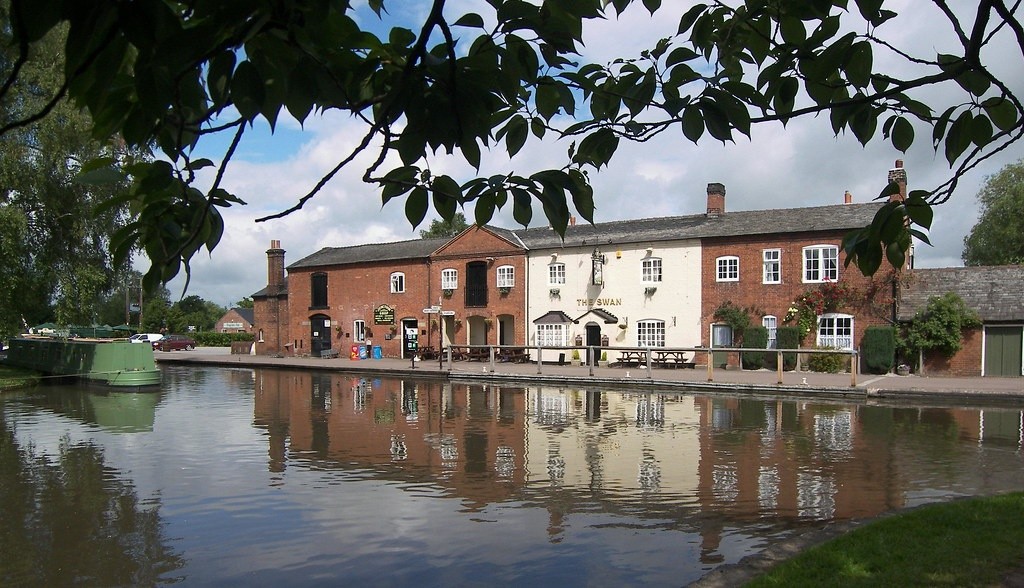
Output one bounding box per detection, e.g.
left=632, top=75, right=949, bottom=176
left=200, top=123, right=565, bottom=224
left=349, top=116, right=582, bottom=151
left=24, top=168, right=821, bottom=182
left=465, top=352, right=490, bottom=357
left=617, top=357, right=647, bottom=361
left=496, top=353, right=530, bottom=358
left=414, top=351, right=439, bottom=355
left=653, top=358, right=688, bottom=362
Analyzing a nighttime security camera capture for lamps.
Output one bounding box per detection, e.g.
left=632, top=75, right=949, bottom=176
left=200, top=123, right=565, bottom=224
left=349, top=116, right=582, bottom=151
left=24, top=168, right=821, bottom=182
left=486, top=257, right=494, bottom=262
left=550, top=253, right=558, bottom=259
left=646, top=247, right=653, bottom=252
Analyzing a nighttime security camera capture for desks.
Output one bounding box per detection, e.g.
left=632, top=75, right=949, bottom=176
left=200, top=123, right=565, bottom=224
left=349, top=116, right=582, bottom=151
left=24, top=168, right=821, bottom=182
left=468, top=348, right=489, bottom=363
left=620, top=352, right=647, bottom=369
left=656, top=351, right=686, bottom=369
left=416, top=346, right=435, bottom=361
left=498, top=348, right=527, bottom=364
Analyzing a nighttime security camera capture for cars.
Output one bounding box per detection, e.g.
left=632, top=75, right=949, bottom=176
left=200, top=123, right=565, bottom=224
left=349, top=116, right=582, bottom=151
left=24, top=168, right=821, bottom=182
left=151, top=335, right=195, bottom=352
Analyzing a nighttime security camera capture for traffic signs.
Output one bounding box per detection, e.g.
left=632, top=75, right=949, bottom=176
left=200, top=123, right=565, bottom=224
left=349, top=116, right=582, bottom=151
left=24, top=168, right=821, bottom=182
left=423, top=305, right=456, bottom=316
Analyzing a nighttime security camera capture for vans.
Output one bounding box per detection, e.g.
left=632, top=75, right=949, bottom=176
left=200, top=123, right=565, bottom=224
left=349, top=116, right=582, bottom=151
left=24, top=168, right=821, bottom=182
left=129, top=334, right=163, bottom=343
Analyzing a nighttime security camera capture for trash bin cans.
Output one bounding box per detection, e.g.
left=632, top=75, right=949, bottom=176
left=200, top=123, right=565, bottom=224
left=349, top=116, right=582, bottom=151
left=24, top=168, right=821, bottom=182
left=374, top=346, right=382, bottom=359
left=351, top=345, right=359, bottom=360
left=359, top=346, right=367, bottom=359
left=559, top=353, right=565, bottom=365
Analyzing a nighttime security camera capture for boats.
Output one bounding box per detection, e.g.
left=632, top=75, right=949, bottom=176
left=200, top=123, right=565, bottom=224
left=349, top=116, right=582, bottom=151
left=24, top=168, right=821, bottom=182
left=1, top=323, right=162, bottom=388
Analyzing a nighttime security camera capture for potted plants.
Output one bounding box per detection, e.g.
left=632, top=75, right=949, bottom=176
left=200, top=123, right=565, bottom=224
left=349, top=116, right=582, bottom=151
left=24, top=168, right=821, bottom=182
left=598, top=352, right=609, bottom=369
left=898, top=365, right=911, bottom=376
left=571, top=349, right=581, bottom=367
left=801, top=362, right=809, bottom=371
